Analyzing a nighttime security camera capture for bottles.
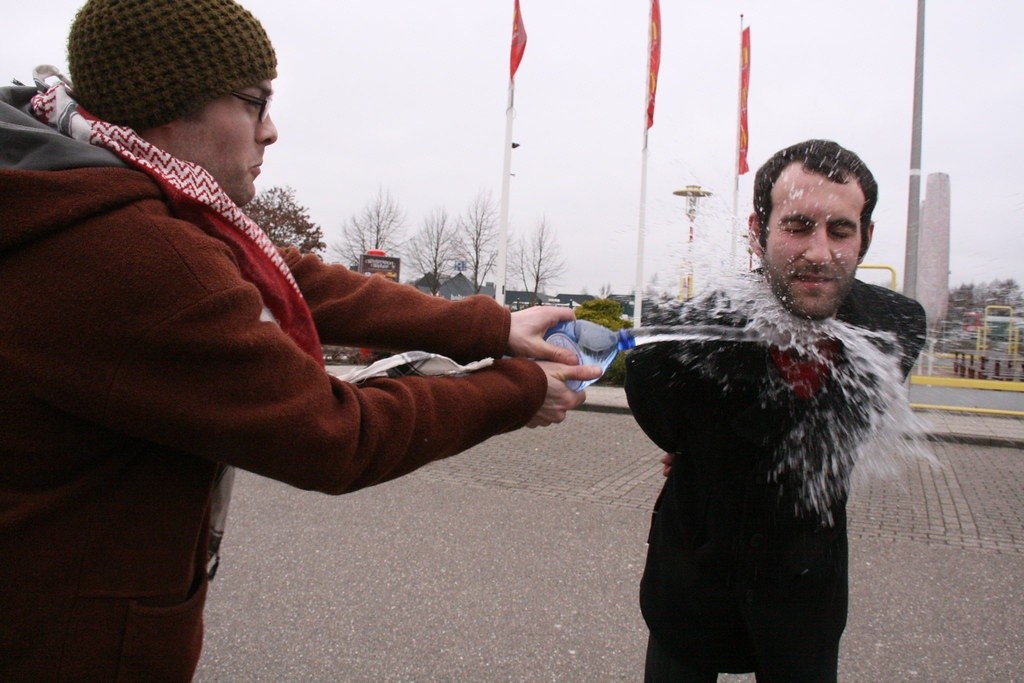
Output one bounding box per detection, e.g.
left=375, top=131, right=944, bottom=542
left=529, top=319, right=637, bottom=392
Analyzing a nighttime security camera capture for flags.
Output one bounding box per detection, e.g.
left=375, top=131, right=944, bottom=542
left=739, top=25, right=751, bottom=172
left=645, top=1, right=662, bottom=130
left=508, top=1, right=530, bottom=79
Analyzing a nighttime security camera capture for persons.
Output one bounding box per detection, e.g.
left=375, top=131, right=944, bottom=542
left=1, top=1, right=606, bottom=683
left=625, top=140, right=928, bottom=683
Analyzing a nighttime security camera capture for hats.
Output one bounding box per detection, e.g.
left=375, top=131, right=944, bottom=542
left=70, top=1, right=277, bottom=129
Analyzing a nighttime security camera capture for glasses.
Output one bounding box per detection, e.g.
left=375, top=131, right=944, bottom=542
left=220, top=86, right=276, bottom=124
